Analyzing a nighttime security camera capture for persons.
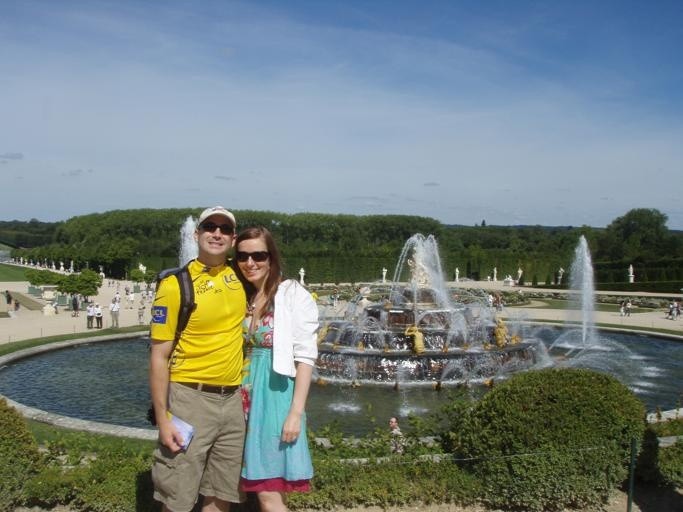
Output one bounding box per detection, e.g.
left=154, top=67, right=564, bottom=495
left=619, top=300, right=624, bottom=315
left=231, top=226, right=318, bottom=511
left=665, top=302, right=672, bottom=320
left=148, top=205, right=252, bottom=511
left=671, top=300, right=678, bottom=321
left=624, top=299, right=631, bottom=317
left=3, top=269, right=153, bottom=332
left=489, top=292, right=493, bottom=311
left=494, top=293, right=503, bottom=312
left=404, top=325, right=426, bottom=353
left=492, top=320, right=508, bottom=347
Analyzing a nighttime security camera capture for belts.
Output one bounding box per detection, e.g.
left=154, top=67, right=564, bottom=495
left=175, top=383, right=239, bottom=395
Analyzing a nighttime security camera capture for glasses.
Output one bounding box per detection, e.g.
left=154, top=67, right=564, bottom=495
left=236, top=251, right=270, bottom=262
left=202, top=221, right=234, bottom=235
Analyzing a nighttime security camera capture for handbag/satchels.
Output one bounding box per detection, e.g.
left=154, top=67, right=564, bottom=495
left=97, top=313, right=101, bottom=315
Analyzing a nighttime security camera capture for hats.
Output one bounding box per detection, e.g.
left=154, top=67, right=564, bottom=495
left=195, top=205, right=236, bottom=229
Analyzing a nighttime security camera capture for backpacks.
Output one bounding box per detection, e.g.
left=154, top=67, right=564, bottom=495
left=146, top=254, right=256, bottom=360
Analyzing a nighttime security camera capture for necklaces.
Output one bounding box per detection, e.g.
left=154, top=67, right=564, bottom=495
left=244, top=290, right=264, bottom=312
